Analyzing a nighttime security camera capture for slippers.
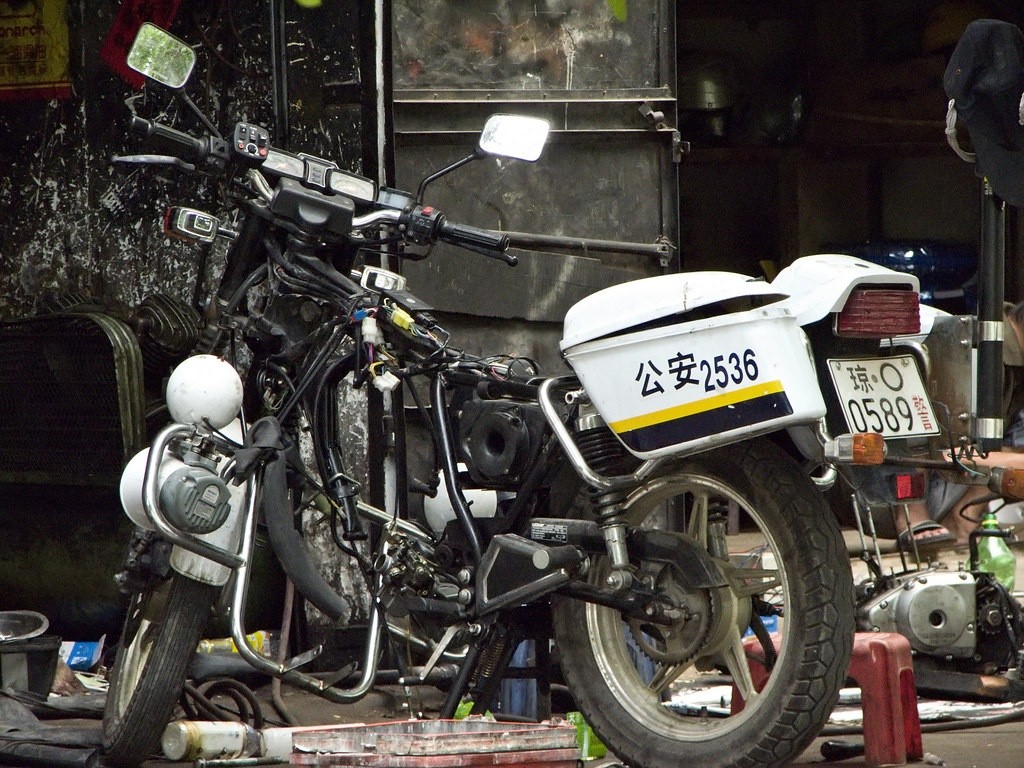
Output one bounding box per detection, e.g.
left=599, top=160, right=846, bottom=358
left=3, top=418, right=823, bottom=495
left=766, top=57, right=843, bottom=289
left=898, top=519, right=955, bottom=550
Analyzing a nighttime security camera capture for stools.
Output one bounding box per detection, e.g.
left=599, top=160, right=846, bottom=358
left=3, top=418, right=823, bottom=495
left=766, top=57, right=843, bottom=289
left=727, top=631, right=924, bottom=768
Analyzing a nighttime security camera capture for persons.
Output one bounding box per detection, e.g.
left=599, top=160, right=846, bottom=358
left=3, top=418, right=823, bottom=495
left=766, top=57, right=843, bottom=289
left=834, top=446, right=990, bottom=549
left=1002, top=300, right=1024, bottom=453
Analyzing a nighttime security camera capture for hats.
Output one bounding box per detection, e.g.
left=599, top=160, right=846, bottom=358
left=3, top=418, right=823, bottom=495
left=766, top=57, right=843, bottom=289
left=945, top=18, right=1024, bottom=209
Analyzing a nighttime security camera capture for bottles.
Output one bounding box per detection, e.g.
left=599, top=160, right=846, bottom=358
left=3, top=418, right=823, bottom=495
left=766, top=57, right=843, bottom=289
left=197, top=628, right=293, bottom=659
left=964, top=513, right=1016, bottom=597
left=161, top=721, right=262, bottom=761
left=453, top=700, right=494, bottom=719
left=574, top=708, right=605, bottom=758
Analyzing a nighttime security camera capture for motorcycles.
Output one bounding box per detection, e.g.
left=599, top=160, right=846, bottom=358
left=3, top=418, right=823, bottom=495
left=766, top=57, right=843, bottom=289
left=67, top=25, right=948, bottom=768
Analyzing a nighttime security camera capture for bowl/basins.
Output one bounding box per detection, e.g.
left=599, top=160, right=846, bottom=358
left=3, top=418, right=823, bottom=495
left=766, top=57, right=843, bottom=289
left=0, top=610, right=49, bottom=642
